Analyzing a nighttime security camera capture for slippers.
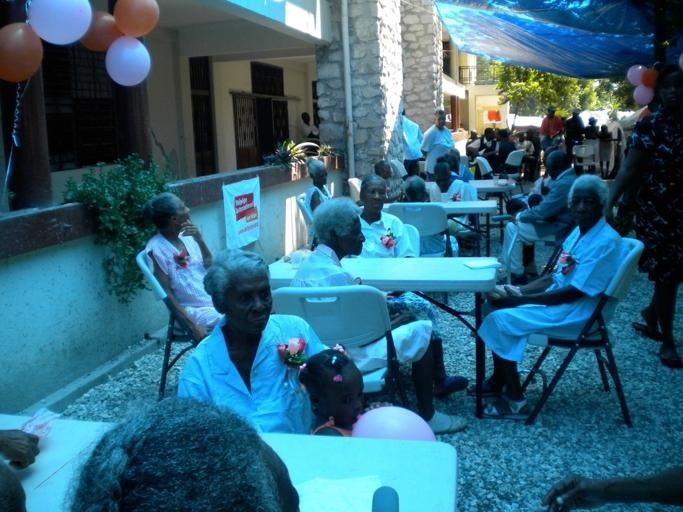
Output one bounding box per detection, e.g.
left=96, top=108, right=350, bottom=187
left=632, top=321, right=662, bottom=340
left=656, top=351, right=683, bottom=368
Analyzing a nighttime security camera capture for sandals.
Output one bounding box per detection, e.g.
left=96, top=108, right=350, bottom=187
left=467, top=376, right=507, bottom=396
left=480, top=397, right=530, bottom=419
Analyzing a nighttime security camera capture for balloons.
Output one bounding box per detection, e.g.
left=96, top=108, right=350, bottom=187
left=627, top=64, right=646, bottom=85
left=0, top=0, right=161, bottom=88
left=642, top=68, right=659, bottom=86
left=632, top=84, right=654, bottom=106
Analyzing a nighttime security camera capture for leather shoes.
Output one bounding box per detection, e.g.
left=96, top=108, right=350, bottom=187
left=435, top=415, right=468, bottom=433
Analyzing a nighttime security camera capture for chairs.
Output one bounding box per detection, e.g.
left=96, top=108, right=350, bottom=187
left=522, top=236, right=645, bottom=429
left=136, top=251, right=204, bottom=400
left=270, top=284, right=412, bottom=411
left=294, top=147, right=645, bottom=258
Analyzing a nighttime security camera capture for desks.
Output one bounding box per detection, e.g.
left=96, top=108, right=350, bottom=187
left=0, top=415, right=459, bottom=512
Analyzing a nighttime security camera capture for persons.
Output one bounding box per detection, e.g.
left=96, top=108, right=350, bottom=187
left=61, top=396, right=302, bottom=511
left=299, top=342, right=367, bottom=437
left=467, top=172, right=628, bottom=418
left=354, top=101, right=633, bottom=284
left=608, top=59, right=683, bottom=369
left=0, top=428, right=41, bottom=512
left=299, top=112, right=320, bottom=157
left=539, top=464, right=683, bottom=512
left=338, top=174, right=469, bottom=400
left=287, top=195, right=469, bottom=435
left=142, top=192, right=227, bottom=343
left=175, top=246, right=327, bottom=434
left=305, top=160, right=334, bottom=216
